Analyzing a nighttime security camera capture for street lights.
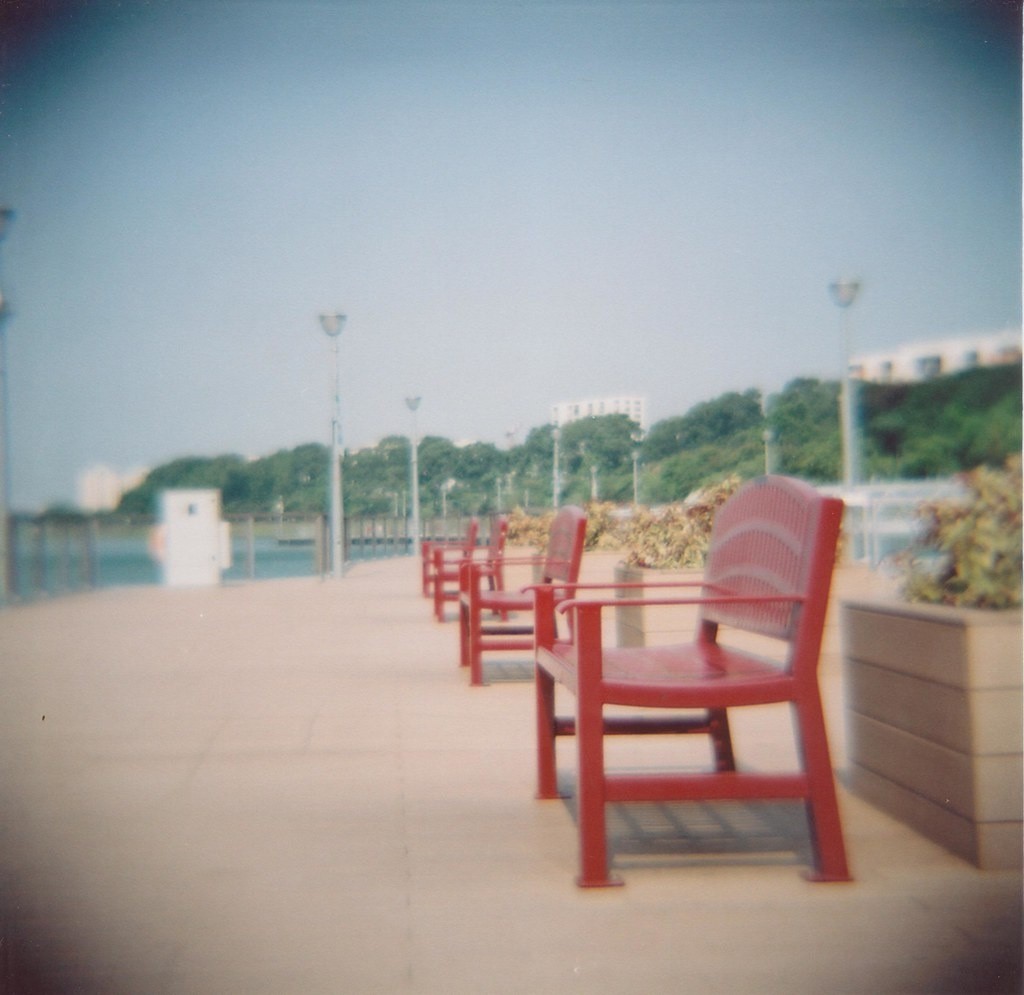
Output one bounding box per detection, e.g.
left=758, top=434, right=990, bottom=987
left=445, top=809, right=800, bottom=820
left=403, top=391, right=424, bottom=559
left=631, top=423, right=647, bottom=505
left=551, top=429, right=562, bottom=506
left=318, top=310, right=350, bottom=575
left=828, top=277, right=860, bottom=492
left=585, top=462, right=599, bottom=508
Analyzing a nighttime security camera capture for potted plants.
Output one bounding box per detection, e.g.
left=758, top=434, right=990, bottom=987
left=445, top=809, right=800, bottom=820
left=503, top=505, right=533, bottom=592
left=839, top=454, right=1023, bottom=872
left=616, top=474, right=741, bottom=648
left=531, top=499, right=622, bottom=614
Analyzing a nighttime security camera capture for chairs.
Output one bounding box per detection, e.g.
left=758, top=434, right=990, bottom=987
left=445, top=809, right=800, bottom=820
left=454, top=505, right=586, bottom=687
left=520, top=475, right=854, bottom=887
left=433, top=514, right=508, bottom=624
left=421, top=518, right=479, bottom=598
